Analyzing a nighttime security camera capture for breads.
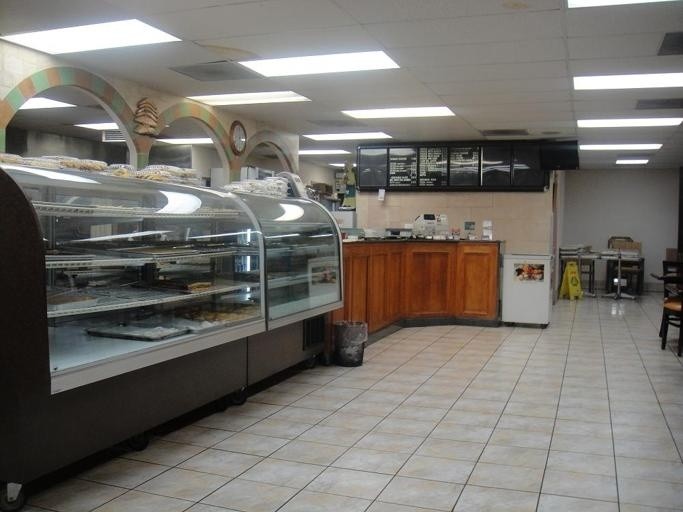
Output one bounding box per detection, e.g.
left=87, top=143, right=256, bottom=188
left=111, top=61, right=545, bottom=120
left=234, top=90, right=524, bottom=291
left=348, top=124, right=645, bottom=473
left=63, top=161, right=162, bottom=182
left=190, top=310, right=238, bottom=321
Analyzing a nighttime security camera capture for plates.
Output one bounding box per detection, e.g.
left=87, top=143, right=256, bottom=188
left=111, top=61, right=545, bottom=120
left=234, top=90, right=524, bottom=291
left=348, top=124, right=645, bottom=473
left=364, top=236, right=381, bottom=240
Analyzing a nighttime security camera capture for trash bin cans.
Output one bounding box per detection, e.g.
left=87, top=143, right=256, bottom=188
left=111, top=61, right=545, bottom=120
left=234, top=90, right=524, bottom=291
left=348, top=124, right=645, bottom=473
left=339, top=321, right=367, bottom=366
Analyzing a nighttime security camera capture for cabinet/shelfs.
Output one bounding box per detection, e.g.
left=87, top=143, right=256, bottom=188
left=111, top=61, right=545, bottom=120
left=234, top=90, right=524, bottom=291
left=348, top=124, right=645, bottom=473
left=222, top=185, right=345, bottom=398
left=0, top=156, right=268, bottom=511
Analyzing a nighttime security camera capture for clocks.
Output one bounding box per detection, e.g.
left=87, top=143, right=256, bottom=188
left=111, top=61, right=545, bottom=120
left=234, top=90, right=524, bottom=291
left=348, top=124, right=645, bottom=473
left=228, top=119, right=248, bottom=156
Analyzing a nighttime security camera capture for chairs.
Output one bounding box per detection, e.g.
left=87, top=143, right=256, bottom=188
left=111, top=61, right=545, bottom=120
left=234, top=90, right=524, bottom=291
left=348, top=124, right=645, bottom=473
left=659, top=259, right=682, bottom=356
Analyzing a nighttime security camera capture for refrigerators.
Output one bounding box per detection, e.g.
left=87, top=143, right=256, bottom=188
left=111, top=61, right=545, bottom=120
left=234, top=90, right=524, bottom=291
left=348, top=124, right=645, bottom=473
left=502, top=254, right=553, bottom=329
